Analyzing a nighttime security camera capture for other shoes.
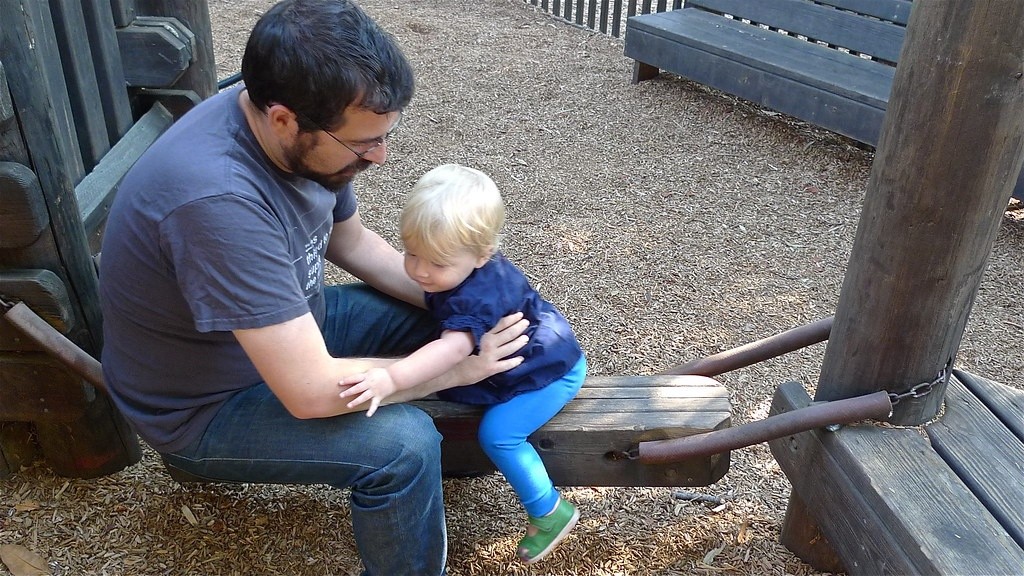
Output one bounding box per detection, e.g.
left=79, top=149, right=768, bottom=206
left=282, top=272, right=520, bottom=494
left=517, top=498, right=580, bottom=564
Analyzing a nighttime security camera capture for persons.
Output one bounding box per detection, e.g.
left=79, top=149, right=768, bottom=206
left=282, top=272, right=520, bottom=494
left=338, top=165, right=588, bottom=563
left=98, top=0, right=530, bottom=576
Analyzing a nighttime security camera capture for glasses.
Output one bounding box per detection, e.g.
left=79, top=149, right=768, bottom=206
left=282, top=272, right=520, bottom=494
left=267, top=102, right=403, bottom=162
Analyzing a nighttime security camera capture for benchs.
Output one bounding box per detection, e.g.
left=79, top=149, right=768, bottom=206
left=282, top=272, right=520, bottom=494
left=623, top=0, right=1024, bottom=203
